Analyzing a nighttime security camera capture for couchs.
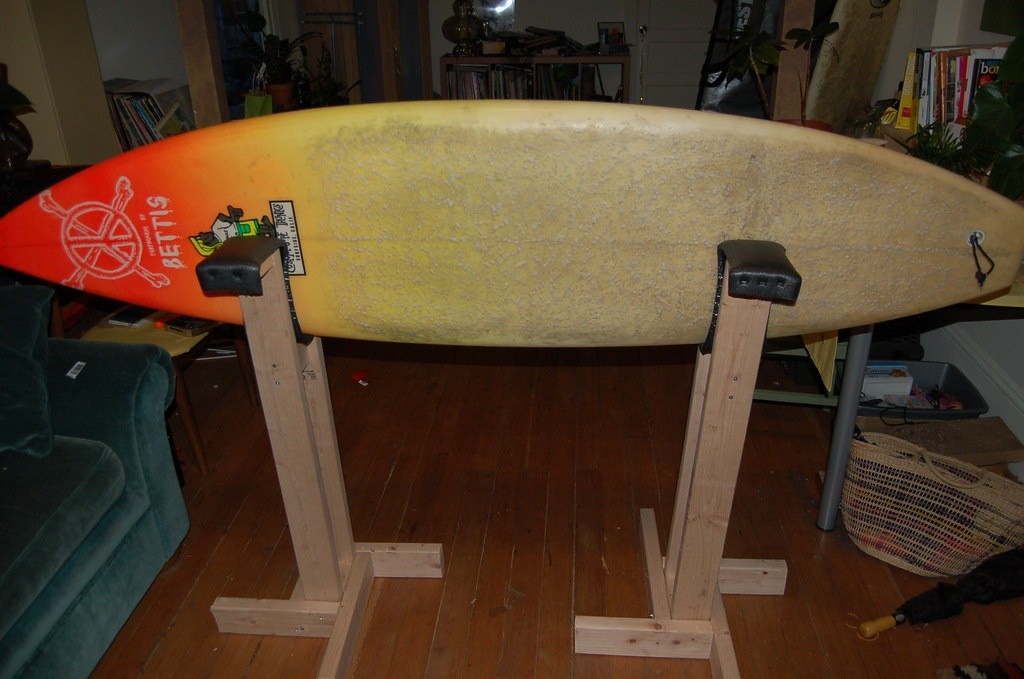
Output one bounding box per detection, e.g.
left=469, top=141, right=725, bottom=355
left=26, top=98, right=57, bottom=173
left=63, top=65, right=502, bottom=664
left=0, top=338, right=191, bottom=679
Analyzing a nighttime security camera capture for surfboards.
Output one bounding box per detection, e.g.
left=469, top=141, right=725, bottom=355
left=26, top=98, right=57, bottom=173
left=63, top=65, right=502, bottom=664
left=0, top=99, right=1023, bottom=348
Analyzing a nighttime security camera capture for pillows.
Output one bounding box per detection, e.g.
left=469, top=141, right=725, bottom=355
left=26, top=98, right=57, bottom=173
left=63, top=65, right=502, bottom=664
left=0, top=284, right=56, bottom=459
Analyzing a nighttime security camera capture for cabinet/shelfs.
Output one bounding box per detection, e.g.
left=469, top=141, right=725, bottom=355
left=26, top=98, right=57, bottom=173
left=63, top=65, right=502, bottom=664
left=439, top=52, right=631, bottom=103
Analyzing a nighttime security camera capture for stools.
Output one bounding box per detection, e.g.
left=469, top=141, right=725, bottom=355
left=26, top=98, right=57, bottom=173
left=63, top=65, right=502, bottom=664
left=880, top=417, right=1024, bottom=476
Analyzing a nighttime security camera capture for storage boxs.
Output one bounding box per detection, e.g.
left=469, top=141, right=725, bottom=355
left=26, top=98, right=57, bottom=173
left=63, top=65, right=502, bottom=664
left=834, top=360, right=989, bottom=420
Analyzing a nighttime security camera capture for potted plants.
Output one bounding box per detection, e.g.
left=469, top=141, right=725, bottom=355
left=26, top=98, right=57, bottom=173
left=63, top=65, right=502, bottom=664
left=701, top=14, right=840, bottom=133
left=223, top=10, right=324, bottom=112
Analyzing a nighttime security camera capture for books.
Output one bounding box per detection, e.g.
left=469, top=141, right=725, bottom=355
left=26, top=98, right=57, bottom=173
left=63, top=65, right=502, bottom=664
left=863, top=43, right=1008, bottom=139
left=104, top=77, right=230, bottom=152
left=447, top=22, right=627, bottom=102
left=108, top=305, right=161, bottom=327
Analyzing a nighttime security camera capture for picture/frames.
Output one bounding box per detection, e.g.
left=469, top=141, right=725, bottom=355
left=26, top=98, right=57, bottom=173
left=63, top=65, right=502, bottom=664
left=597, top=22, right=626, bottom=45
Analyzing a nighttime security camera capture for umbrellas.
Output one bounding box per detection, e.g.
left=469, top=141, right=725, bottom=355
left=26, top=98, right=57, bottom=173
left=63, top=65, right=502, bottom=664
left=858, top=542, right=1024, bottom=638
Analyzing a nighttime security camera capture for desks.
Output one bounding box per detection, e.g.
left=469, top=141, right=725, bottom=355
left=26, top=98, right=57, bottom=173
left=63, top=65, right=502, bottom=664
left=80, top=305, right=261, bottom=479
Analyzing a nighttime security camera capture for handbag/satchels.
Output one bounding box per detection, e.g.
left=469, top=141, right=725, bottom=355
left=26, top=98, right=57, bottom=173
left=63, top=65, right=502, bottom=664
left=244, top=71, right=272, bottom=118
left=839, top=431, right=1023, bottom=581
